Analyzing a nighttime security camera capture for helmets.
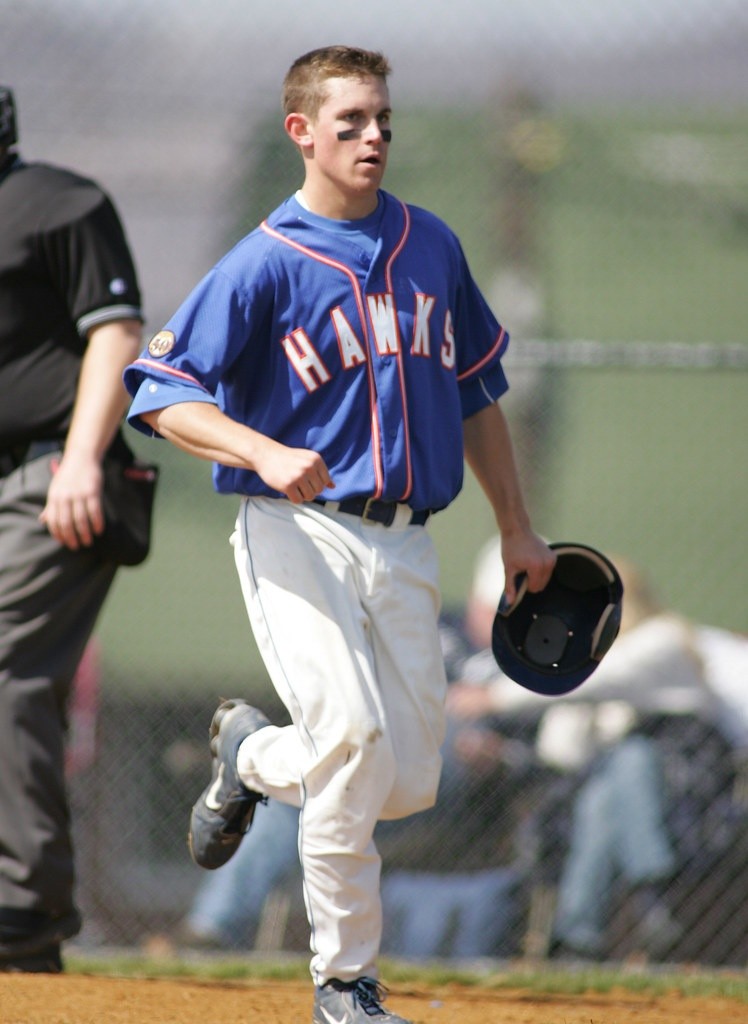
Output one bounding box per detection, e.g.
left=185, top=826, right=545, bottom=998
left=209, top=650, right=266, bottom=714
left=493, top=543, right=623, bottom=697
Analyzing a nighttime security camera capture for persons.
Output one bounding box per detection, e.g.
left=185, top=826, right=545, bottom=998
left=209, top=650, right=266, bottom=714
left=147, top=522, right=748, bottom=960
left=0, top=83, right=146, bottom=975
left=121, top=47, right=557, bottom=1024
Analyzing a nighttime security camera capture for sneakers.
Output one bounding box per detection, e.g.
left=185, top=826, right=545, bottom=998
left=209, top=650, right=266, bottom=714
left=313, top=978, right=417, bottom=1024
left=189, top=699, right=272, bottom=870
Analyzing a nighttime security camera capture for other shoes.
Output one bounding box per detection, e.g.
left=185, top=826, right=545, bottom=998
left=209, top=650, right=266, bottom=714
left=547, top=934, right=617, bottom=964
left=0, top=943, right=64, bottom=974
left=635, top=904, right=699, bottom=961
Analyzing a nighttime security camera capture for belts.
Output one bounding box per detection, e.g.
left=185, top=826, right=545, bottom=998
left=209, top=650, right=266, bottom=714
left=311, top=491, right=433, bottom=526
